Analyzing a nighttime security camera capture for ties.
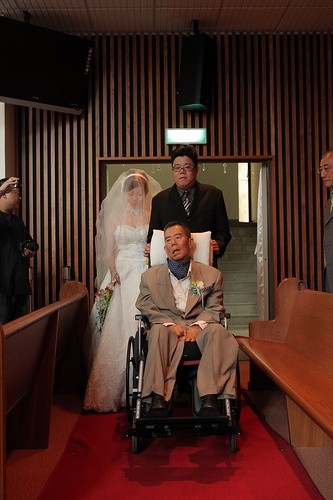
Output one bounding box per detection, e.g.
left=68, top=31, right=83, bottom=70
left=183, top=191, right=192, bottom=217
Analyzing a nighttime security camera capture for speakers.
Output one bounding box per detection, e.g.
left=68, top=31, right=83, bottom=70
left=176, top=33, right=214, bottom=109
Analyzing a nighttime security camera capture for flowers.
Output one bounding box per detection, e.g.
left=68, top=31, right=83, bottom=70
left=191, top=280, right=205, bottom=297
left=94, top=279, right=117, bottom=333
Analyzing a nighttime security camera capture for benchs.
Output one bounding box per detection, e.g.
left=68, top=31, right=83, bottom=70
left=234, top=277, right=333, bottom=500
left=0, top=280, right=90, bottom=500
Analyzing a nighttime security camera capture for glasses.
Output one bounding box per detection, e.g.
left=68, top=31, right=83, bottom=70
left=317, top=166, right=333, bottom=174
left=172, top=164, right=195, bottom=172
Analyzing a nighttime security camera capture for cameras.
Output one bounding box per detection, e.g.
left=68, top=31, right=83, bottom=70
left=18, top=240, right=39, bottom=252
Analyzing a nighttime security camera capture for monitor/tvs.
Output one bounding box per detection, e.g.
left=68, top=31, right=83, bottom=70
left=0, top=14, right=96, bottom=115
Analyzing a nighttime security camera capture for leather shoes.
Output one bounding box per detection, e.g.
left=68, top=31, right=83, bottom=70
left=152, top=394, right=167, bottom=410
left=202, top=399, right=216, bottom=411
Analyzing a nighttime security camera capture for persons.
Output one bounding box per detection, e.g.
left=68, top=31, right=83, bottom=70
left=143, top=146, right=232, bottom=259
left=135, top=220, right=238, bottom=421
left=0, top=175, right=36, bottom=324
left=317, top=149, right=333, bottom=293
left=84, top=169, right=163, bottom=411
